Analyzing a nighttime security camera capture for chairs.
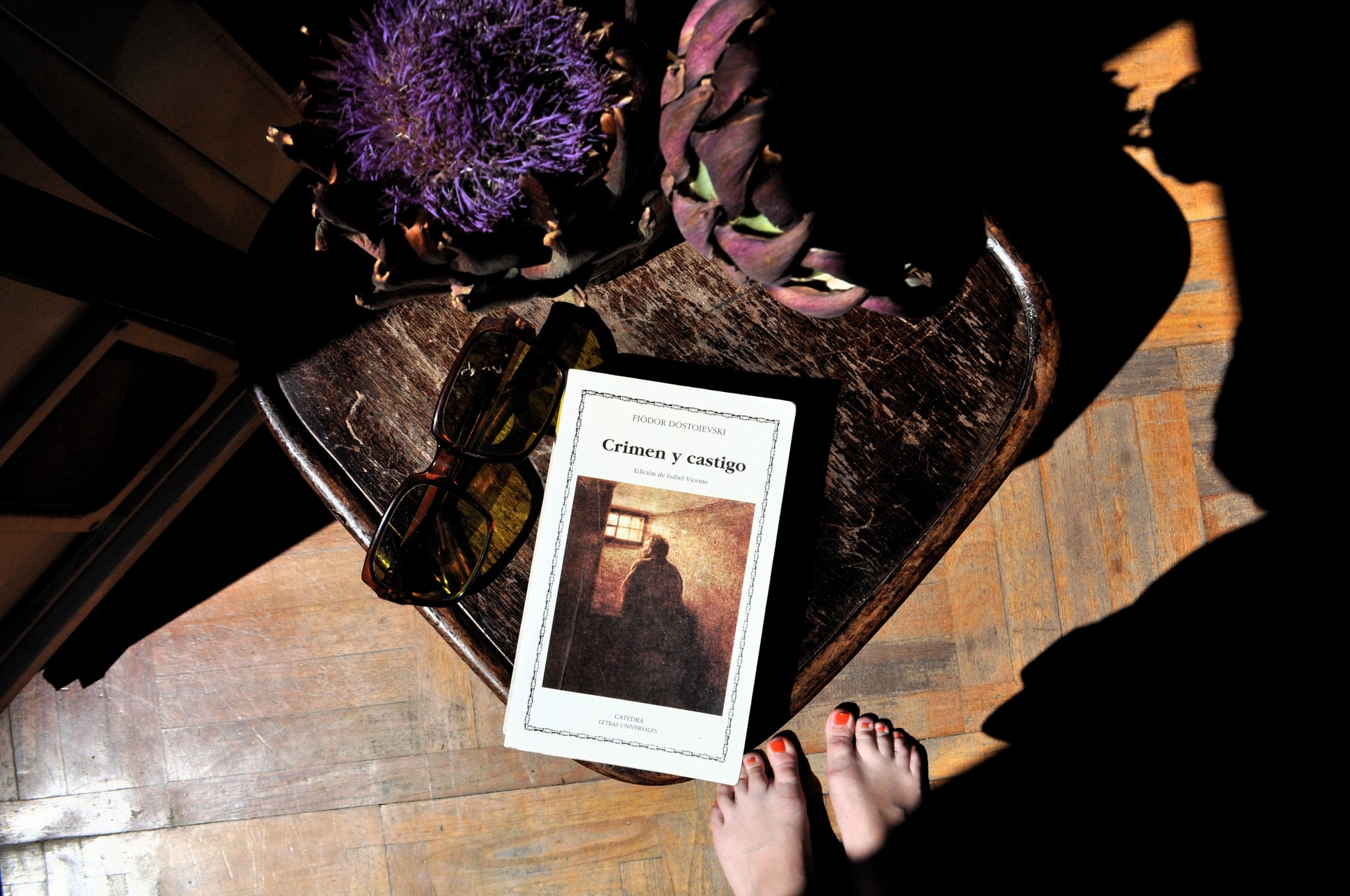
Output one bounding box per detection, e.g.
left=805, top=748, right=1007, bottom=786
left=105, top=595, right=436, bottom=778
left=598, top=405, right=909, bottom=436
left=0, top=0, right=1057, bottom=785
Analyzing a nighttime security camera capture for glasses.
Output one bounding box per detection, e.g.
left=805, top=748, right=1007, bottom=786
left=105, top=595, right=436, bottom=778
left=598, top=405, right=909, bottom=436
left=362, top=313, right=566, bottom=606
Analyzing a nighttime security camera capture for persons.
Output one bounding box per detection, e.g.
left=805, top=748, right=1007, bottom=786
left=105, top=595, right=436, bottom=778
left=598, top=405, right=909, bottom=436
left=698, top=471, right=1350, bottom=896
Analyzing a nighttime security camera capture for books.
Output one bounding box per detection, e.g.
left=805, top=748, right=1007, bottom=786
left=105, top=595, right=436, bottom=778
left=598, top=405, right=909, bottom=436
left=498, top=368, right=797, bottom=786
